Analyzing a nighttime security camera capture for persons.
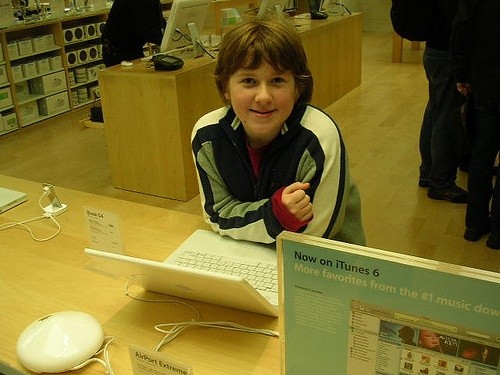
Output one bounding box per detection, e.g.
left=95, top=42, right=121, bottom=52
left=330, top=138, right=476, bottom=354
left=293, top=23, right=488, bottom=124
left=191, top=15, right=365, bottom=247
left=398, top=326, right=416, bottom=346
left=102, top=1, right=167, bottom=67
left=420, top=330, right=441, bottom=352
left=481, top=347, right=489, bottom=363
left=390, top=0, right=500, bottom=250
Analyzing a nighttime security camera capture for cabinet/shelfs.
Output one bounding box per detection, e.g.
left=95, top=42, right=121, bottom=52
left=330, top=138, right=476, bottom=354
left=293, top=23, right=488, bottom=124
left=2, top=19, right=72, bottom=130
left=58, top=10, right=109, bottom=109
left=0, top=30, right=20, bottom=136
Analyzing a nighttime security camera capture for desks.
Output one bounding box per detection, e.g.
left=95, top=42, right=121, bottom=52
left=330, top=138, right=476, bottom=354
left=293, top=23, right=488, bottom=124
left=99, top=11, right=362, bottom=202
left=0, top=174, right=280, bottom=375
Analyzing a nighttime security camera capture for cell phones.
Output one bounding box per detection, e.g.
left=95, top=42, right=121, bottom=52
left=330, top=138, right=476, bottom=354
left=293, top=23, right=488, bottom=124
left=42, top=183, right=63, bottom=208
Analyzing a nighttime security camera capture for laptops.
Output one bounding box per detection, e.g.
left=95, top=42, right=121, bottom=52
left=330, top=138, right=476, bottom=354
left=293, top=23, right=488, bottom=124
left=84, top=228, right=279, bottom=317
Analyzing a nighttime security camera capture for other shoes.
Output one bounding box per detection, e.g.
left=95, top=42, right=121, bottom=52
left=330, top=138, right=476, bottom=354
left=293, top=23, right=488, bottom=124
left=464, top=217, right=492, bottom=242
left=428, top=185, right=469, bottom=204
left=418, top=170, right=433, bottom=188
left=487, top=226, right=500, bottom=249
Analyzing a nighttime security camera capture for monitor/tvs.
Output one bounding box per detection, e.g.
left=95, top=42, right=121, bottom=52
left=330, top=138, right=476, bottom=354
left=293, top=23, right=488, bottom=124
left=257, top=0, right=287, bottom=18
left=160, top=0, right=210, bottom=59
